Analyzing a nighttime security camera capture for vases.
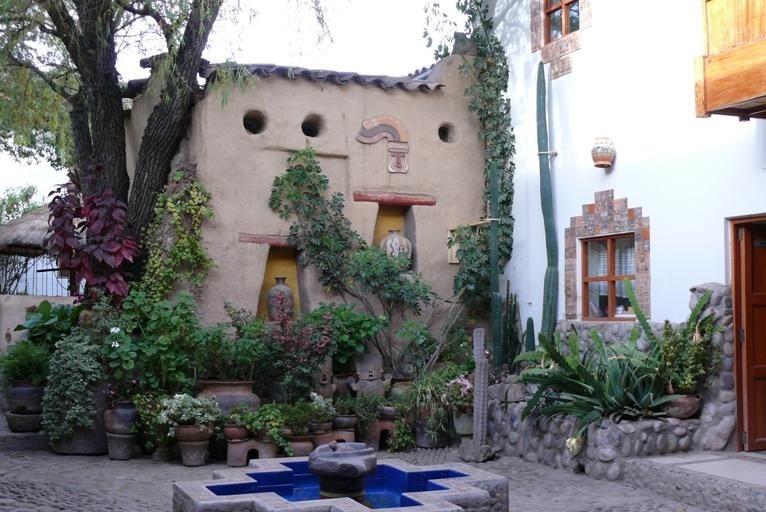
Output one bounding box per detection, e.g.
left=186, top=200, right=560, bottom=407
left=452, top=411, right=474, bottom=435
left=269, top=275, right=293, bottom=322
left=380, top=228, right=413, bottom=272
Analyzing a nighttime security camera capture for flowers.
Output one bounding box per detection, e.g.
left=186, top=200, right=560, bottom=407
left=440, top=375, right=474, bottom=414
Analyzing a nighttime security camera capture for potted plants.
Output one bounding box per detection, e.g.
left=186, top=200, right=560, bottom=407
left=2, top=304, right=448, bottom=466
left=658, top=298, right=726, bottom=420
